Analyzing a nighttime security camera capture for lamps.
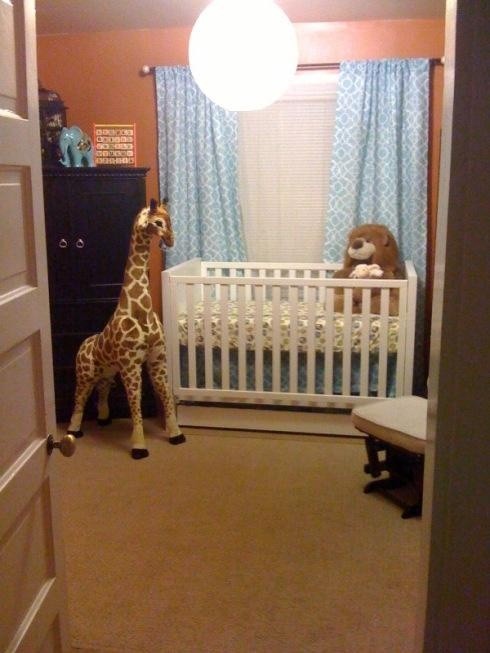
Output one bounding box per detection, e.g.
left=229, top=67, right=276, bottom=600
left=187, top=0, right=299, bottom=112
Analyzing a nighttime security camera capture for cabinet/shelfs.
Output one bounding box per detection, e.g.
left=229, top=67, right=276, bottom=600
left=43, top=164, right=150, bottom=422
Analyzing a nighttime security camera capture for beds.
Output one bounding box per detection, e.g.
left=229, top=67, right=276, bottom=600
left=163, top=253, right=417, bottom=440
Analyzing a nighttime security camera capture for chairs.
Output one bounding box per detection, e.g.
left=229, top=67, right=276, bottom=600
left=353, top=390, right=427, bottom=518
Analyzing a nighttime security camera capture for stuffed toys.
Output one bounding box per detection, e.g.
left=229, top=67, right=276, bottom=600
left=61, top=194, right=186, bottom=463
left=348, top=262, right=382, bottom=280
left=322, top=223, right=403, bottom=319
